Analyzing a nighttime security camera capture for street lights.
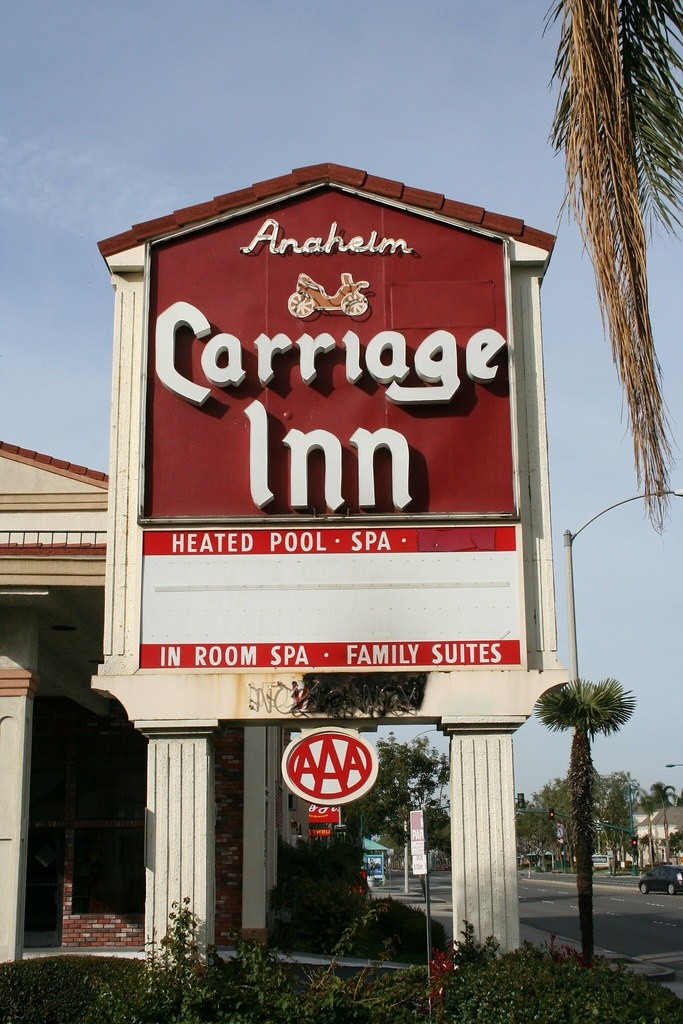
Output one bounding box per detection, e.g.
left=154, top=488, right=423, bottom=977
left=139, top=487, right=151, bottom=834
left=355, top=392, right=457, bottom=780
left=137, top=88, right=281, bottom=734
left=560, top=485, right=682, bottom=699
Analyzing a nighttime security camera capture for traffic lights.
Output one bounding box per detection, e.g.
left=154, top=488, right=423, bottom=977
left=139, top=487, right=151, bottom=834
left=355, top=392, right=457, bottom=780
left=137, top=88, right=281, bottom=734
left=631, top=837, right=638, bottom=847
left=517, top=793, right=525, bottom=808
left=548, top=808, right=556, bottom=822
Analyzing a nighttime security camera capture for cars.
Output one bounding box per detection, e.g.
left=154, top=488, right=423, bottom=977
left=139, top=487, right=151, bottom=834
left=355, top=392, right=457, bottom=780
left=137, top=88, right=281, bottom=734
left=638, top=865, right=683, bottom=895
